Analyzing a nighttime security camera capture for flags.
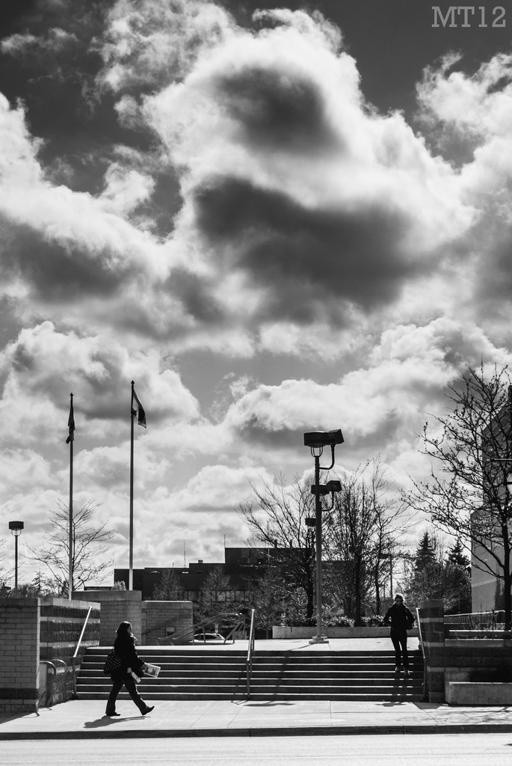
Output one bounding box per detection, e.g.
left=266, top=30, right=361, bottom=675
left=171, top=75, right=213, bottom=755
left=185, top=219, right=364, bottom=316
left=131, top=386, right=148, bottom=431
left=66, top=396, right=76, bottom=444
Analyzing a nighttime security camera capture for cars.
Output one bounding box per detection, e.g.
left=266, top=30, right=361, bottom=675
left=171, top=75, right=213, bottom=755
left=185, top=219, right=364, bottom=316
left=195, top=633, right=225, bottom=641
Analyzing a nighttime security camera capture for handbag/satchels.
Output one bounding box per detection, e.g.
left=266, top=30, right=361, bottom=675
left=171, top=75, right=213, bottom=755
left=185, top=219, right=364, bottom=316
left=103, top=651, right=124, bottom=676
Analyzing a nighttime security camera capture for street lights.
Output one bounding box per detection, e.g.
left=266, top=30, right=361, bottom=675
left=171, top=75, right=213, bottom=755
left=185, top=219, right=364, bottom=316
left=8, top=519, right=26, bottom=593
left=301, top=427, right=346, bottom=646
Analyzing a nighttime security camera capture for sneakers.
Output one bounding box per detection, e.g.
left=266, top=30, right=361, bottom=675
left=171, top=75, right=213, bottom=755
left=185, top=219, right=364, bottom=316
left=394, top=665, right=411, bottom=678
left=106, top=710, right=121, bottom=717
left=139, top=705, right=154, bottom=716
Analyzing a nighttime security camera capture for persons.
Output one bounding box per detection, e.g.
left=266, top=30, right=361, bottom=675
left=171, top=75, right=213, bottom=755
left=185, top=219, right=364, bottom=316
left=106, top=621, right=154, bottom=717
left=382, top=594, right=415, bottom=677
